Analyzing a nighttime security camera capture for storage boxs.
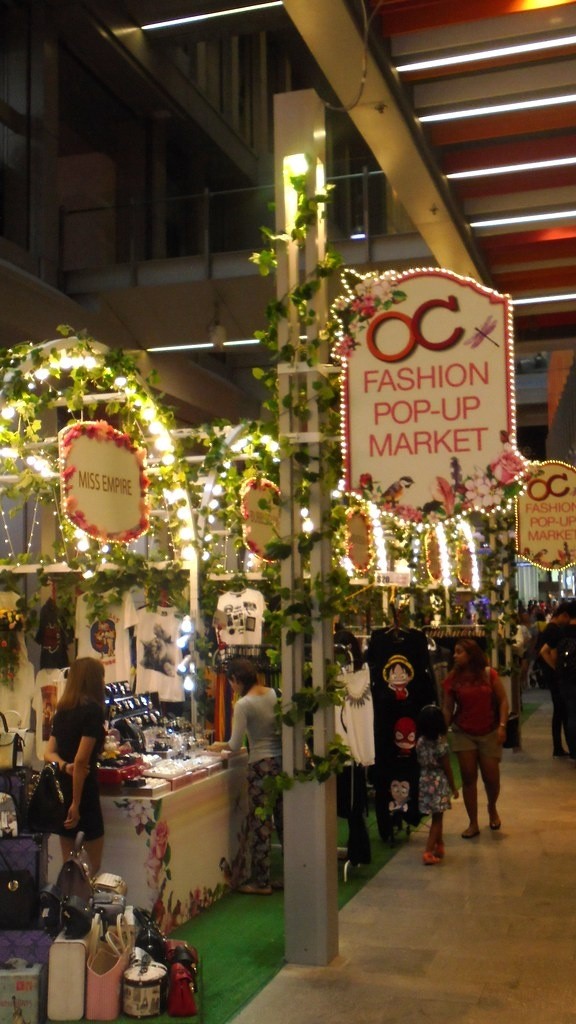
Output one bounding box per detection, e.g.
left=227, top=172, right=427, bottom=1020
left=0, top=962, right=42, bottom=1024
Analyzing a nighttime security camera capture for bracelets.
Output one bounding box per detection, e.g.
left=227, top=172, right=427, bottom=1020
left=61, top=762, right=68, bottom=775
left=500, top=723, right=507, bottom=727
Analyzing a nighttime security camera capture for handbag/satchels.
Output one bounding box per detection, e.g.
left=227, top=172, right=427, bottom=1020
left=0, top=708, right=200, bottom=1024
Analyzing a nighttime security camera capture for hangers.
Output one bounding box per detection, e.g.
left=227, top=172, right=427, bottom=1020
left=386, top=622, right=506, bottom=638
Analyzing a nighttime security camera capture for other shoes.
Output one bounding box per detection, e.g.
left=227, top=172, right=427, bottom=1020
left=239, top=885, right=271, bottom=895
left=487, top=805, right=501, bottom=830
left=422, top=841, right=445, bottom=865
left=461, top=823, right=481, bottom=839
left=553, top=748, right=576, bottom=760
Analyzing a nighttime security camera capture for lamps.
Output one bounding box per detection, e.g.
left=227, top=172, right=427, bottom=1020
left=174, top=614, right=194, bottom=674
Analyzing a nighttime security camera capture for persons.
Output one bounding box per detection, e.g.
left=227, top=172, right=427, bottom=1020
left=209, top=658, right=315, bottom=896
left=501, top=591, right=576, bottom=760
left=44, top=657, right=107, bottom=880
left=333, top=623, right=510, bottom=866
